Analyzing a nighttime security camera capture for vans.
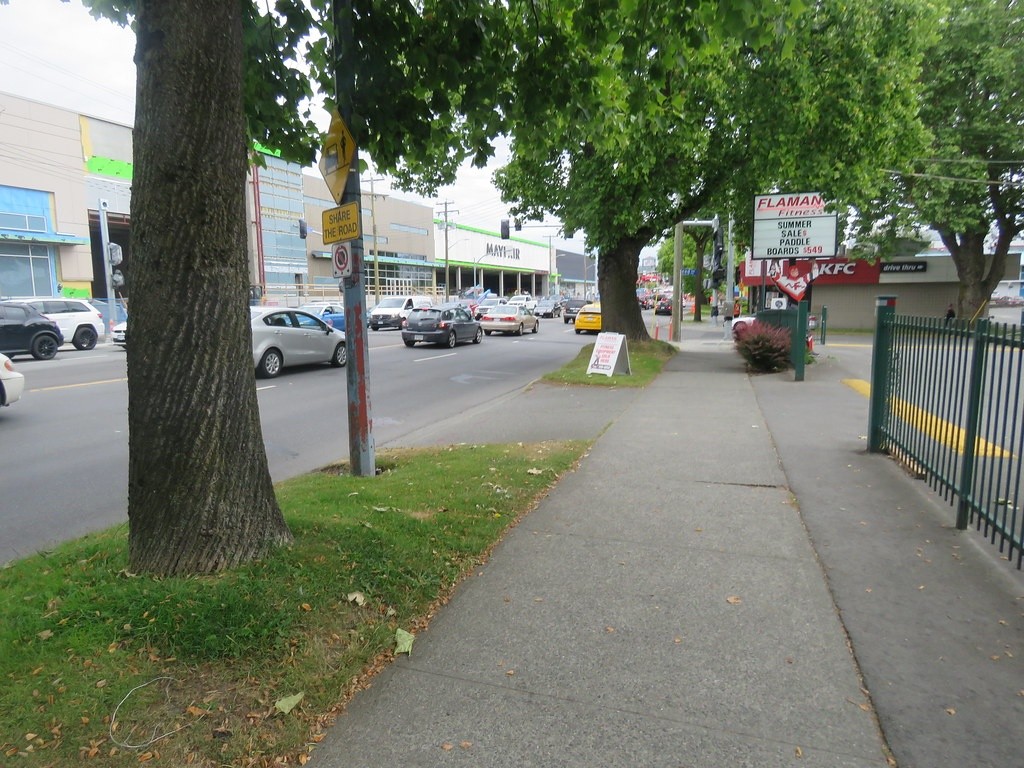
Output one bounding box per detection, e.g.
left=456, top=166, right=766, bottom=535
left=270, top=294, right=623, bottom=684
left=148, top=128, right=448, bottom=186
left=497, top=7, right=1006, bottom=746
left=369, top=294, right=435, bottom=331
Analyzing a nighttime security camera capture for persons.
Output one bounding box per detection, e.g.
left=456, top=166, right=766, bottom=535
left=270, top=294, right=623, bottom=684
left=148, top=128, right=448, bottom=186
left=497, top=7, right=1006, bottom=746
left=946, top=304, right=955, bottom=319
left=734, top=300, right=740, bottom=317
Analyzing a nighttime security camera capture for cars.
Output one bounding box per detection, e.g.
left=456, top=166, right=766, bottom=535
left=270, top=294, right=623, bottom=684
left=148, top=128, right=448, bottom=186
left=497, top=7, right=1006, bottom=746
left=574, top=303, right=602, bottom=334
left=0, top=352, right=25, bottom=407
left=655, top=300, right=672, bottom=316
left=731, top=311, right=759, bottom=336
left=447, top=286, right=601, bottom=318
left=0, top=299, right=65, bottom=361
left=534, top=300, right=562, bottom=318
left=401, top=301, right=484, bottom=348
left=249, top=304, right=345, bottom=379
left=636, top=284, right=687, bottom=310
left=112, top=322, right=128, bottom=350
left=3, top=296, right=105, bottom=351
left=995, top=295, right=1024, bottom=306
left=280, top=302, right=346, bottom=333
left=479, top=303, right=541, bottom=336
left=990, top=291, right=1000, bottom=301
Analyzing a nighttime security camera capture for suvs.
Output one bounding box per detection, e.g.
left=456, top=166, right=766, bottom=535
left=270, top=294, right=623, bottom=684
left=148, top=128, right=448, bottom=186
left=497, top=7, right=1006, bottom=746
left=563, top=298, right=593, bottom=324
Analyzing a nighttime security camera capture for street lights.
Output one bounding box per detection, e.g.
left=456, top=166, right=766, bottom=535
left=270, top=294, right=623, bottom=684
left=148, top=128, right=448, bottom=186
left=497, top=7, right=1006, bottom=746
left=445, top=237, right=469, bottom=302
left=548, top=254, right=565, bottom=296
left=473, top=248, right=515, bottom=288
left=583, top=264, right=598, bottom=300
